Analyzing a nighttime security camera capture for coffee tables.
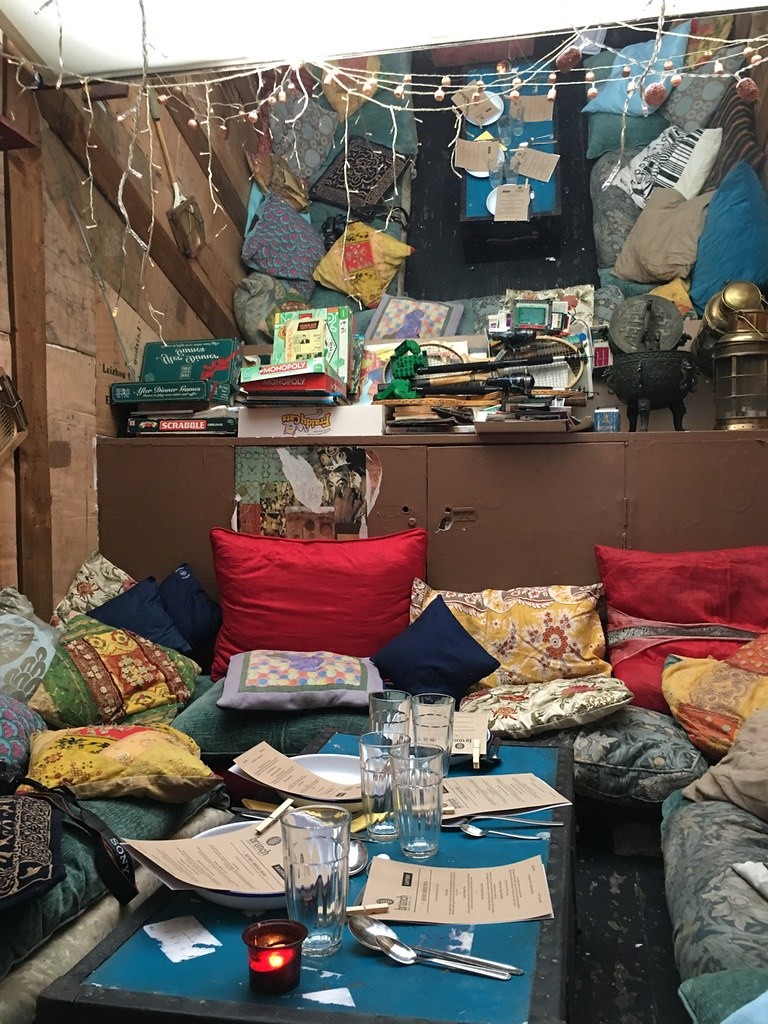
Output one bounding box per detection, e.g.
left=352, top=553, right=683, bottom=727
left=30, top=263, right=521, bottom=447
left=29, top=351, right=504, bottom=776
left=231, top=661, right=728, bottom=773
left=36, top=729, right=575, bottom=1024
left=459, top=61, right=562, bottom=252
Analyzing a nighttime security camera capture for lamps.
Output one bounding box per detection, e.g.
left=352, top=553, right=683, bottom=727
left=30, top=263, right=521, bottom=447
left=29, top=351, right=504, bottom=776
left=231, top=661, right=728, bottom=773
left=689, top=280, right=768, bottom=375
left=712, top=307, right=768, bottom=433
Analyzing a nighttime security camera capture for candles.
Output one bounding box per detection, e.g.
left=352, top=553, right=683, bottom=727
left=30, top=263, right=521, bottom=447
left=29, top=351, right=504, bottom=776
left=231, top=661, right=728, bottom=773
left=241, top=918, right=308, bottom=996
left=496, top=60, right=510, bottom=72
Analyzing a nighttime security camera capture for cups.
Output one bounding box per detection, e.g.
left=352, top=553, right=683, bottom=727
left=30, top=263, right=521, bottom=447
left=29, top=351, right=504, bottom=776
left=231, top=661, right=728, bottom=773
left=359, top=731, right=411, bottom=841
left=280, top=805, right=350, bottom=958
left=388, top=743, right=444, bottom=859
left=412, top=693, right=455, bottom=778
left=369, top=690, right=412, bottom=735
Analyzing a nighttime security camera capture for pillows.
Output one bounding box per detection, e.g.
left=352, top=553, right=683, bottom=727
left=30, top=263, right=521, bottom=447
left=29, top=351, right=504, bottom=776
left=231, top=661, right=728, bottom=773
left=0, top=523, right=768, bottom=1024
left=234, top=16, right=768, bottom=353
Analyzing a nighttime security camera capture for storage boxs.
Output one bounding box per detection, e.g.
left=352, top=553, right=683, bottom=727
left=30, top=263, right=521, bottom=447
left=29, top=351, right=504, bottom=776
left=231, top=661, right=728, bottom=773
left=110, top=305, right=382, bottom=437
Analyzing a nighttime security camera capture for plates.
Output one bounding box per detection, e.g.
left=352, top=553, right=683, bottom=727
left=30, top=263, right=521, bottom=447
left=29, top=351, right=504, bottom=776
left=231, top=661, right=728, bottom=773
left=190, top=821, right=286, bottom=912
left=486, top=728, right=491, bottom=742
left=276, top=754, right=363, bottom=814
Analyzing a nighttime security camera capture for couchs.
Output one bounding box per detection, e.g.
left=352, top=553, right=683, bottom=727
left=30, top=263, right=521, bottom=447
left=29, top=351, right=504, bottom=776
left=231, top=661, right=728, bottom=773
left=140, top=672, right=707, bottom=802
left=0, top=769, right=237, bottom=1024
left=662, top=724, right=768, bottom=1024
left=389, top=284, right=625, bottom=339
left=243, top=54, right=417, bottom=309
left=584, top=46, right=685, bottom=300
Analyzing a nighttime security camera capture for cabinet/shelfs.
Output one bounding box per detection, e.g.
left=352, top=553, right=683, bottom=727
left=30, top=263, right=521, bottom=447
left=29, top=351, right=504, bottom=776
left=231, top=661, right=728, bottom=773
left=245, top=441, right=624, bottom=593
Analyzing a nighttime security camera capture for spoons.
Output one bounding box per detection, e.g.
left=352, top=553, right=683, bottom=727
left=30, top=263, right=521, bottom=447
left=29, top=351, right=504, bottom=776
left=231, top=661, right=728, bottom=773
left=491, top=737, right=502, bottom=761
left=375, top=935, right=511, bottom=980
left=479, top=734, right=494, bottom=765
left=349, top=840, right=368, bottom=875
left=440, top=816, right=564, bottom=827
left=460, top=824, right=541, bottom=839
left=353, top=854, right=391, bottom=906
left=348, top=915, right=524, bottom=974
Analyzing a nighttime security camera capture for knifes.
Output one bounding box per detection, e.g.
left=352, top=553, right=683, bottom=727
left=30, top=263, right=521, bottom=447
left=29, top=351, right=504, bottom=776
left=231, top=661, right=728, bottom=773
left=230, top=804, right=273, bottom=816
left=241, top=811, right=380, bottom=843
